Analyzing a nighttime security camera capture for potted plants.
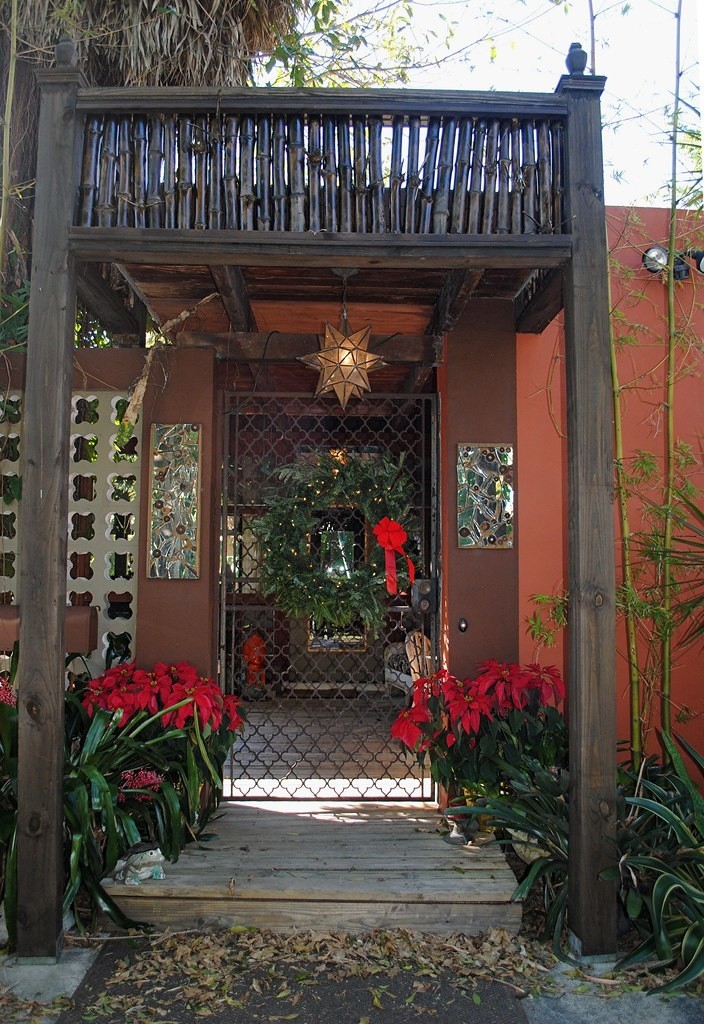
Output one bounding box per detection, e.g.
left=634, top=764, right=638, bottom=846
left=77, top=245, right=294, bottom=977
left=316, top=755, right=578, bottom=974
left=0, top=692, right=223, bottom=948
left=443, top=740, right=677, bottom=945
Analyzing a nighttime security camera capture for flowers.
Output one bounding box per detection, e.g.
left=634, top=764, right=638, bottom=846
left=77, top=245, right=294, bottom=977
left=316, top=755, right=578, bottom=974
left=82, top=657, right=246, bottom=775
left=391, top=659, right=566, bottom=827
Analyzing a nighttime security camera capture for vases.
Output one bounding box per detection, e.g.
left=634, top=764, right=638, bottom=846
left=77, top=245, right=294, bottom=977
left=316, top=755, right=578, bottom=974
left=144, top=779, right=211, bottom=843
left=505, top=828, right=551, bottom=865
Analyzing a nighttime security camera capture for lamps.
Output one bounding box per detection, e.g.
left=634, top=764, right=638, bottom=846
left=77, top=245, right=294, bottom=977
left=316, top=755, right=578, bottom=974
left=295, top=268, right=391, bottom=411
left=642, top=246, right=704, bottom=280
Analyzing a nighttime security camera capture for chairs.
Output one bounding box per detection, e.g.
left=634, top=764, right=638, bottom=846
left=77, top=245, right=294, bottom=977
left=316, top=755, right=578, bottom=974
left=405, top=631, right=431, bottom=708
